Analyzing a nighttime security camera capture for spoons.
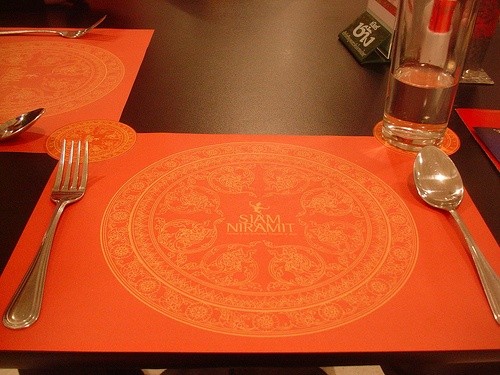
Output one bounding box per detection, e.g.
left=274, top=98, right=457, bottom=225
left=0, top=108, right=45, bottom=141
left=414, top=145, right=500, bottom=327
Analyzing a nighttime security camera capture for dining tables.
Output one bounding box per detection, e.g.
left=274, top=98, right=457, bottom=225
left=0, top=1, right=499, bottom=369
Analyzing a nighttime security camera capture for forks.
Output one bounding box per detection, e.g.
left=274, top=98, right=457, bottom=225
left=0, top=14, right=106, bottom=39
left=1, top=139, right=88, bottom=330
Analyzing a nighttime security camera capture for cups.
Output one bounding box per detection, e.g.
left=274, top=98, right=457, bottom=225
left=381, top=0, right=480, bottom=152
